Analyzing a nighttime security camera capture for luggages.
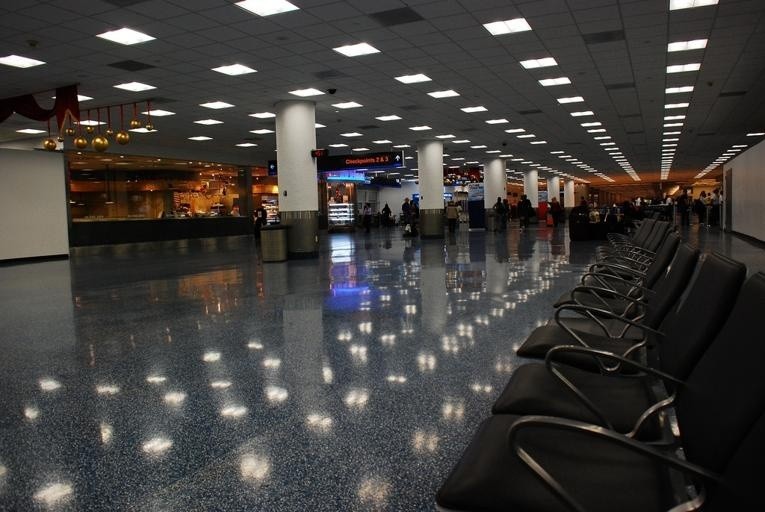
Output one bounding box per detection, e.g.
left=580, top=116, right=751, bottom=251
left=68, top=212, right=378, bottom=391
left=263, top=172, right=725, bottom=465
left=546, top=214, right=554, bottom=226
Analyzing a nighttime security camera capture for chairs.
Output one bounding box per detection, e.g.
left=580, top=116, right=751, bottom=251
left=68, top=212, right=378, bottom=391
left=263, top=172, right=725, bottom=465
left=515, top=211, right=748, bottom=372
left=437, top=412, right=764, bottom=510
left=489, top=271, right=764, bottom=448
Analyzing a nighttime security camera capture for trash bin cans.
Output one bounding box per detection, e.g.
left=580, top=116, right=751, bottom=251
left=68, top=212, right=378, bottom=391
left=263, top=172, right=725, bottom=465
left=420, top=209, right=446, bottom=240
left=260, top=225, right=288, bottom=263
left=529, top=207, right=540, bottom=224
left=570, top=206, right=589, bottom=241
left=704, top=206, right=714, bottom=228
left=484, top=208, right=500, bottom=232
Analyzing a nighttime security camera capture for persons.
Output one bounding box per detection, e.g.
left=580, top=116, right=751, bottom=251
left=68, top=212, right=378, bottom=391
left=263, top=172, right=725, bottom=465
left=666, top=188, right=723, bottom=231
left=548, top=198, right=562, bottom=228
left=362, top=197, right=418, bottom=233
left=445, top=201, right=463, bottom=233
left=580, top=196, right=588, bottom=206
left=254, top=207, right=267, bottom=248
left=494, top=194, right=531, bottom=233
